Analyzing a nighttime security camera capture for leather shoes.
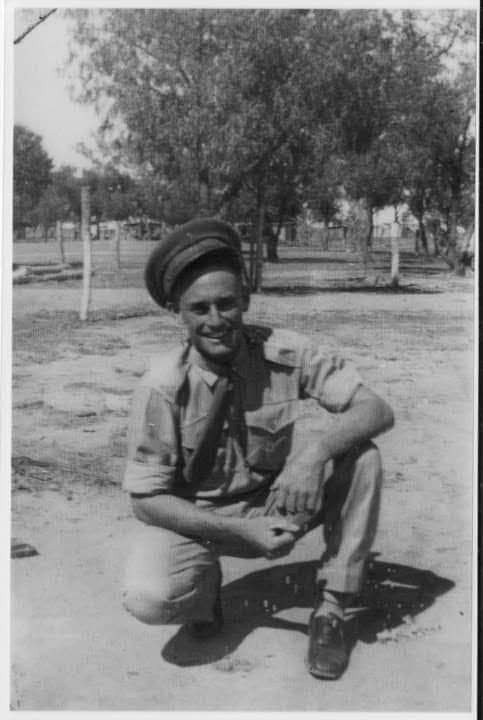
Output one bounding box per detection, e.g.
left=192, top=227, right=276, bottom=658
left=185, top=561, right=223, bottom=640
left=304, top=611, right=349, bottom=679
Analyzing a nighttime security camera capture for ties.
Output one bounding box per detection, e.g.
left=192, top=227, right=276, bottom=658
left=179, top=376, right=235, bottom=500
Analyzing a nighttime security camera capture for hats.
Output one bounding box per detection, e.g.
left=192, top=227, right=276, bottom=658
left=144, top=220, right=242, bottom=306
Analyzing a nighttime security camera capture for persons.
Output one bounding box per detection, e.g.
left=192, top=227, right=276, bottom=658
left=125, top=220, right=394, bottom=679
